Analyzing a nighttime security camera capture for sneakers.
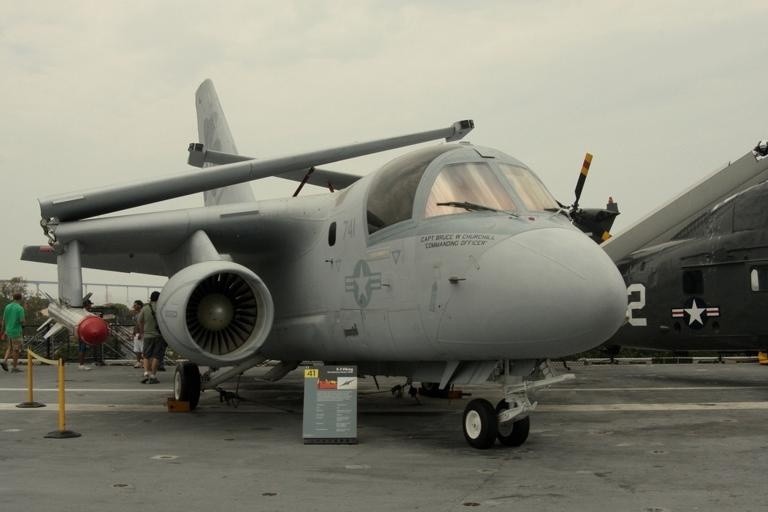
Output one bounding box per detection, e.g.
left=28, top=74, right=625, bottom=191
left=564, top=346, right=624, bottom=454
left=149, top=376, right=161, bottom=384
left=134, top=361, right=145, bottom=369
left=10, top=367, right=24, bottom=375
left=0, top=362, right=9, bottom=374
left=77, top=364, right=92, bottom=371
left=139, top=375, right=149, bottom=384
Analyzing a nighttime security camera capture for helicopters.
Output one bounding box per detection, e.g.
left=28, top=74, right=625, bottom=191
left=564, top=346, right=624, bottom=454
left=547, top=139, right=766, bottom=371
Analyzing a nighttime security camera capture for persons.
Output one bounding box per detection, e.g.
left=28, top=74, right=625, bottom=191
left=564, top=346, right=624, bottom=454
left=136, top=291, right=166, bottom=384
left=158, top=356, right=165, bottom=371
left=131, top=300, right=144, bottom=368
left=0, top=293, right=27, bottom=373
left=79, top=299, right=94, bottom=369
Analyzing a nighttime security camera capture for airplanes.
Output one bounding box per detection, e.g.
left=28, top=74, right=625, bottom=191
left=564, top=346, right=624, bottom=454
left=16, top=80, right=629, bottom=446
left=338, top=378, right=355, bottom=388
